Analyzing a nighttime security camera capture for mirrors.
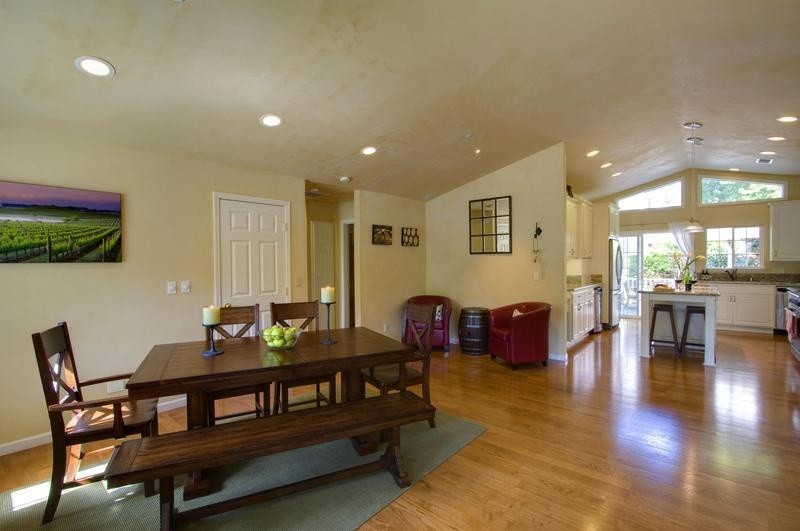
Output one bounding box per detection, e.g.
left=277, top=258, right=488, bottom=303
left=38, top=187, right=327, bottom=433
left=469, top=196, right=512, bottom=254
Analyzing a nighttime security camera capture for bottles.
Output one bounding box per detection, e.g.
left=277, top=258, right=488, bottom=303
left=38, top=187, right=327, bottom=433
left=701, top=270, right=705, bottom=280
left=705, top=269, right=709, bottom=281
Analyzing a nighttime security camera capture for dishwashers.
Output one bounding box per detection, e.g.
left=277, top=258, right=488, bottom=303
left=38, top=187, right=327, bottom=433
left=773, top=286, right=796, bottom=336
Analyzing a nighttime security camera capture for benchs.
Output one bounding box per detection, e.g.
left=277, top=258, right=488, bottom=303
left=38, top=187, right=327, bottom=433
left=104, top=390, right=436, bottom=531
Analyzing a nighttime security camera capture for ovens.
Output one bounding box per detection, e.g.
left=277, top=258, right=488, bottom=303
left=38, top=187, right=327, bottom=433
left=785, top=291, right=800, bottom=358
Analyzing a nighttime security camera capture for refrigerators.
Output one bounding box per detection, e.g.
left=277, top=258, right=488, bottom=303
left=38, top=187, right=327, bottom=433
left=609, top=238, right=623, bottom=329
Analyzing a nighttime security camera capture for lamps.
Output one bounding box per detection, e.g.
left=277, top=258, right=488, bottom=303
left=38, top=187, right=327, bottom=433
left=683, top=122, right=705, bottom=233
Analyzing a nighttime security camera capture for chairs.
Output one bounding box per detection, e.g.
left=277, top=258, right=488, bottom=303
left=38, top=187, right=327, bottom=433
left=206, top=304, right=269, bottom=425
left=406, top=295, right=452, bottom=358
left=488, top=302, right=552, bottom=369
left=360, top=303, right=437, bottom=442
left=32, top=322, right=158, bottom=525
left=271, top=301, right=337, bottom=416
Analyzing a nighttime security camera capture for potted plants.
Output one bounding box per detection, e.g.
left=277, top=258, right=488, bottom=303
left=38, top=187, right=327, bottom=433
left=684, top=274, right=692, bottom=291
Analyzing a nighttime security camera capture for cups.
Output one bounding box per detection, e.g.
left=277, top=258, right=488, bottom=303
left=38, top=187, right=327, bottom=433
left=678, top=284, right=684, bottom=292
left=694, top=274, right=697, bottom=280
left=710, top=276, right=712, bottom=281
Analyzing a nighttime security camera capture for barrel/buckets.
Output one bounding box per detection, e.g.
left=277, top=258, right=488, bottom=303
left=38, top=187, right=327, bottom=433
left=458, top=307, right=489, bottom=355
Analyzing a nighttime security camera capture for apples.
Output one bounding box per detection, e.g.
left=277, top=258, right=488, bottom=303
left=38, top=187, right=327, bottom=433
left=263, top=325, right=297, bottom=348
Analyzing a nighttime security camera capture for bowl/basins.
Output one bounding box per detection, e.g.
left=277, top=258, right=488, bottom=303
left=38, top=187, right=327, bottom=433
left=259, top=327, right=303, bottom=350
left=720, top=273, right=727, bottom=280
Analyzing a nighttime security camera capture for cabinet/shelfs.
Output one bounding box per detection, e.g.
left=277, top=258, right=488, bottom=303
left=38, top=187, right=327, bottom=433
left=566, top=196, right=579, bottom=260
left=578, top=196, right=593, bottom=259
left=566, top=288, right=594, bottom=349
left=767, top=200, right=800, bottom=261
left=692, top=285, right=777, bottom=334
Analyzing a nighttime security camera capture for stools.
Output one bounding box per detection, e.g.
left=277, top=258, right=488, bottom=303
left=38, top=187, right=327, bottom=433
left=681, top=306, right=705, bottom=356
left=649, top=304, right=679, bottom=356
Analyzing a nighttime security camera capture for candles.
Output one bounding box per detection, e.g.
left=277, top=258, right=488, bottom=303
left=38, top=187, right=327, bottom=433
left=203, top=305, right=221, bottom=325
left=321, top=285, right=336, bottom=303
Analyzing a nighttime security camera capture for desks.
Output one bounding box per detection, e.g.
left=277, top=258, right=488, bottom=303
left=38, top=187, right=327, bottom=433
left=459, top=307, right=489, bottom=355
left=128, top=326, right=417, bottom=501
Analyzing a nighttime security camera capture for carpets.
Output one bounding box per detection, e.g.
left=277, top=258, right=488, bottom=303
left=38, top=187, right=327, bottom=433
left=1, top=383, right=489, bottom=531
